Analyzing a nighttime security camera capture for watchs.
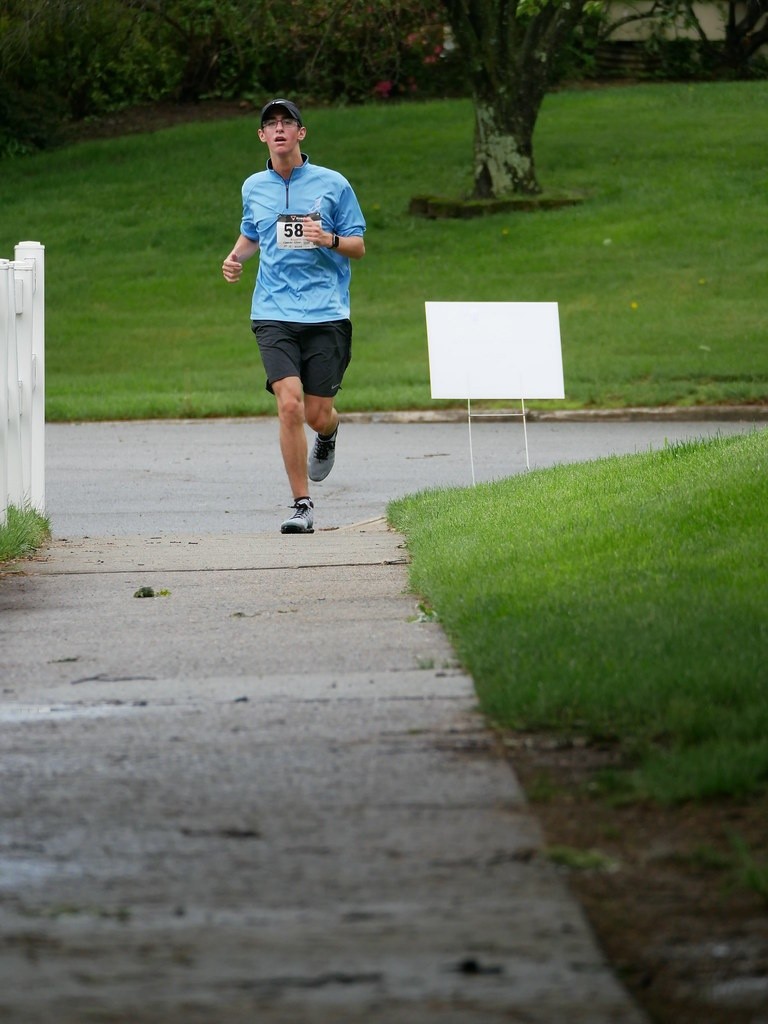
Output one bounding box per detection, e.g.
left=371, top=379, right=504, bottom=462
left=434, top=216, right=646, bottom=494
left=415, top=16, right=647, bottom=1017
left=326, top=233, right=339, bottom=250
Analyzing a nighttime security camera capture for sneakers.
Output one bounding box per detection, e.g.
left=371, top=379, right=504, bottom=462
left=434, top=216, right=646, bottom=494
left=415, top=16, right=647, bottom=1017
left=307, top=421, right=339, bottom=482
left=281, top=497, right=314, bottom=534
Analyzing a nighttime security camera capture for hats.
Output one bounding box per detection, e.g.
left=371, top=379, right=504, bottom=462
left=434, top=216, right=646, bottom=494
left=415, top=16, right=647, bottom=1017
left=261, top=99, right=302, bottom=127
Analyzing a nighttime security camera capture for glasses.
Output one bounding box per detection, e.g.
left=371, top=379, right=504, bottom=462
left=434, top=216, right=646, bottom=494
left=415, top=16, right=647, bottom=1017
left=263, top=118, right=301, bottom=129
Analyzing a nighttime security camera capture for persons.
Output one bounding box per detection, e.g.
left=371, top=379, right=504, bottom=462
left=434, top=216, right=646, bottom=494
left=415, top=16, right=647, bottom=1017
left=223, top=98, right=367, bottom=534
